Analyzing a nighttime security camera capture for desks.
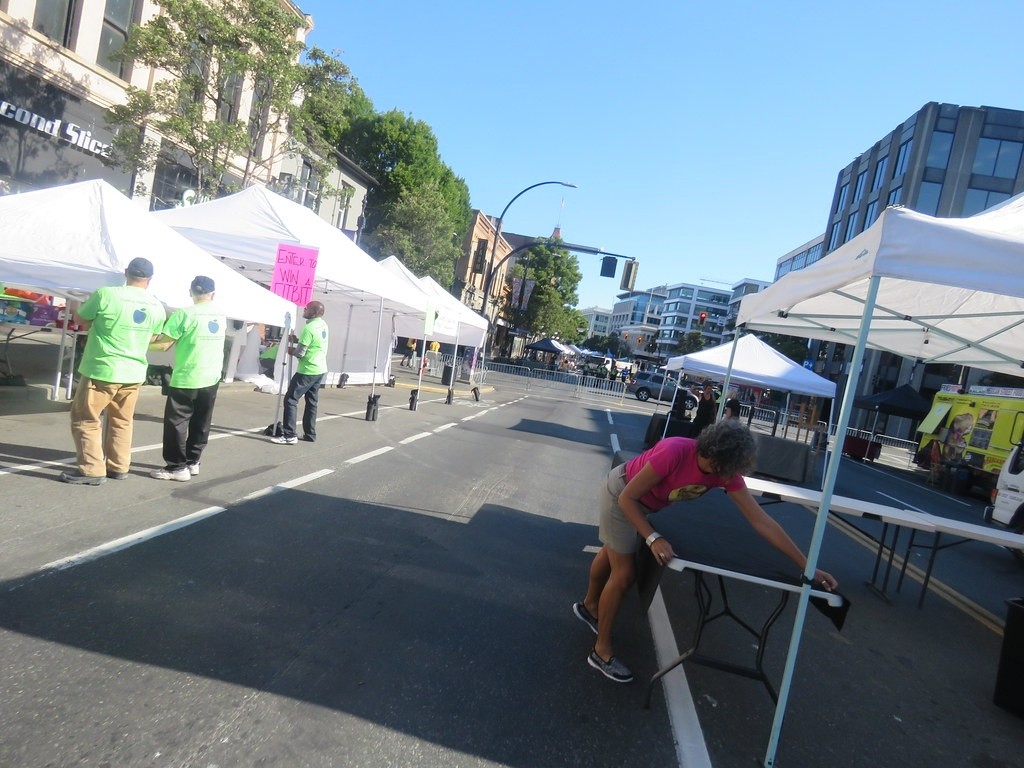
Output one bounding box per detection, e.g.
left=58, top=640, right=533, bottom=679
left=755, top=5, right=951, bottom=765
left=0, top=298, right=89, bottom=375
left=609, top=431, right=1024, bottom=709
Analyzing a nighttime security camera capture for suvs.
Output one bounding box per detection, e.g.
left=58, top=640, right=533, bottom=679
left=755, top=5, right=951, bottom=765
left=629, top=370, right=699, bottom=410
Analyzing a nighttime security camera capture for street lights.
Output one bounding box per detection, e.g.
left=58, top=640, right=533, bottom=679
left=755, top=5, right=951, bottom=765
left=480, top=180, right=580, bottom=318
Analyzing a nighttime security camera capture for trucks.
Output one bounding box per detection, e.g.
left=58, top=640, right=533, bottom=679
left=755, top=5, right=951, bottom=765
left=982, top=411, right=1024, bottom=560
left=583, top=355, right=634, bottom=379
left=912, top=382, right=1024, bottom=495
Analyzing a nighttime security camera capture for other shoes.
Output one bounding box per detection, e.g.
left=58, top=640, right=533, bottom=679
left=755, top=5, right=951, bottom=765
left=107, top=470, right=128, bottom=479
left=60, top=469, right=106, bottom=485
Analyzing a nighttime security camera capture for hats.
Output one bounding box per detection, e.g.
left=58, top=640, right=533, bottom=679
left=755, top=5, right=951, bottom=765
left=129, top=258, right=153, bottom=278
left=191, top=276, right=215, bottom=295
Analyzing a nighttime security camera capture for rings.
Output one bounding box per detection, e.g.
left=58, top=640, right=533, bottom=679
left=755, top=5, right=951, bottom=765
left=660, top=553, right=664, bottom=557
left=822, top=581, right=826, bottom=584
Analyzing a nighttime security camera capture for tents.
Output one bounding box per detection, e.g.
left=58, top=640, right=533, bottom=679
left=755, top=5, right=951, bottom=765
left=852, top=383, right=931, bottom=468
left=716, top=192, right=1024, bottom=768
left=525, top=338, right=582, bottom=355
left=0, top=179, right=297, bottom=437
left=656, top=334, right=837, bottom=491
left=149, top=183, right=489, bottom=398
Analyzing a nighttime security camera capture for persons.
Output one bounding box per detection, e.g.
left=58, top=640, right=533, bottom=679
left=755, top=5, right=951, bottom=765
left=61, top=256, right=166, bottom=486
left=724, top=391, right=740, bottom=420
left=596, top=363, right=608, bottom=387
left=430, top=341, right=440, bottom=352
left=582, top=362, right=589, bottom=384
left=689, top=381, right=716, bottom=424
left=549, top=355, right=568, bottom=368
left=573, top=417, right=838, bottom=683
left=259, top=345, right=279, bottom=379
left=750, top=394, right=756, bottom=405
left=148, top=276, right=226, bottom=481
left=715, top=385, right=723, bottom=411
left=610, top=366, right=619, bottom=389
left=400, top=338, right=418, bottom=368
left=621, top=366, right=628, bottom=382
left=271, top=301, right=329, bottom=443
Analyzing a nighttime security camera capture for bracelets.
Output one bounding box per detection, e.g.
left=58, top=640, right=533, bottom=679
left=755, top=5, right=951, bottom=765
left=646, top=532, right=663, bottom=547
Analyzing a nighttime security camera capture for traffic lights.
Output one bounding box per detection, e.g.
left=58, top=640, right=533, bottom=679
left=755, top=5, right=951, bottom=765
left=625, top=333, right=629, bottom=339
left=697, top=311, right=708, bottom=327
left=638, top=336, right=641, bottom=344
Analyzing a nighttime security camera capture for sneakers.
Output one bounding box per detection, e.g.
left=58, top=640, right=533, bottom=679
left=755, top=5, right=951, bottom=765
left=587, top=647, right=633, bottom=683
left=150, top=466, right=191, bottom=481
left=188, top=461, right=200, bottom=475
left=573, top=600, right=598, bottom=635
left=270, top=435, right=298, bottom=444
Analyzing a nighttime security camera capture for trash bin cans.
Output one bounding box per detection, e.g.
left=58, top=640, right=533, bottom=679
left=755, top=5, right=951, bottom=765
left=992, top=597, right=1024, bottom=719
left=441, top=364, right=457, bottom=386
